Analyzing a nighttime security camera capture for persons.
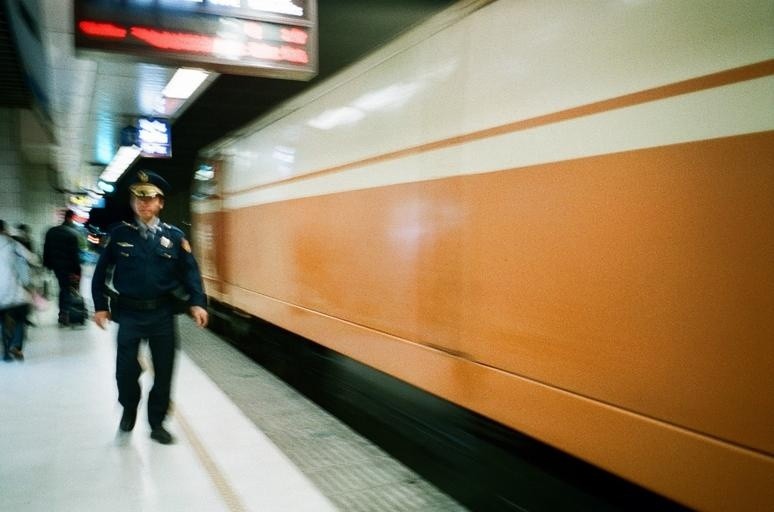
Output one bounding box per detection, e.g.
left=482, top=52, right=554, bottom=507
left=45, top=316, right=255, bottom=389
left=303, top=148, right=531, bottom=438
left=0, top=220, right=42, bottom=361
left=92, top=169, right=209, bottom=445
left=10, top=224, right=37, bottom=326
left=44, top=210, right=88, bottom=327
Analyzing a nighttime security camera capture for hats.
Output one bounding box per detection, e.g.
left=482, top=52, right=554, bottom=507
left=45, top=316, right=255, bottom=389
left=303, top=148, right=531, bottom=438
left=128, top=169, right=169, bottom=199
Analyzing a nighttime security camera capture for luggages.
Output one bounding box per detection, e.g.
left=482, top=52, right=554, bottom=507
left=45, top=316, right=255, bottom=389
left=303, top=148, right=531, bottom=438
left=57, top=294, right=89, bottom=327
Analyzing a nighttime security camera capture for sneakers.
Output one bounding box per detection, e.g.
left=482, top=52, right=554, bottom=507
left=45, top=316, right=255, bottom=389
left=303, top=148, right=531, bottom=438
left=150, top=426, right=172, bottom=444
left=120, top=403, right=136, bottom=433
left=1, top=346, right=25, bottom=361
